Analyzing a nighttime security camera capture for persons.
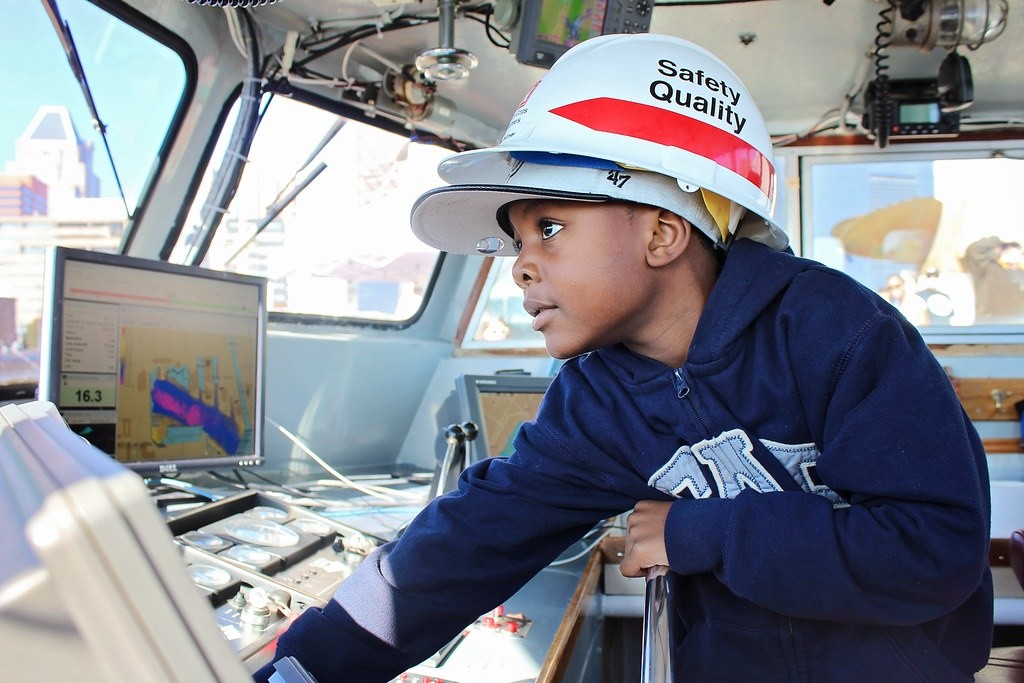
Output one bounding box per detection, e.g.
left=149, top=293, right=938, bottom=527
left=887, top=274, right=931, bottom=326
left=253, top=34, right=995, bottom=682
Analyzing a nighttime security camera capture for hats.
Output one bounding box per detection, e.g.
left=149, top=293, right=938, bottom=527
left=410, top=159, right=718, bottom=258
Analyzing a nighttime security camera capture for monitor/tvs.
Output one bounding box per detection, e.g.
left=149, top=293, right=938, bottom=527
left=40, top=247, right=266, bottom=470
left=458, top=375, right=556, bottom=465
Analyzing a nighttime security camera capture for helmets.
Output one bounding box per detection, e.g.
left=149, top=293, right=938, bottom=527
left=436, top=33, right=790, bottom=252
